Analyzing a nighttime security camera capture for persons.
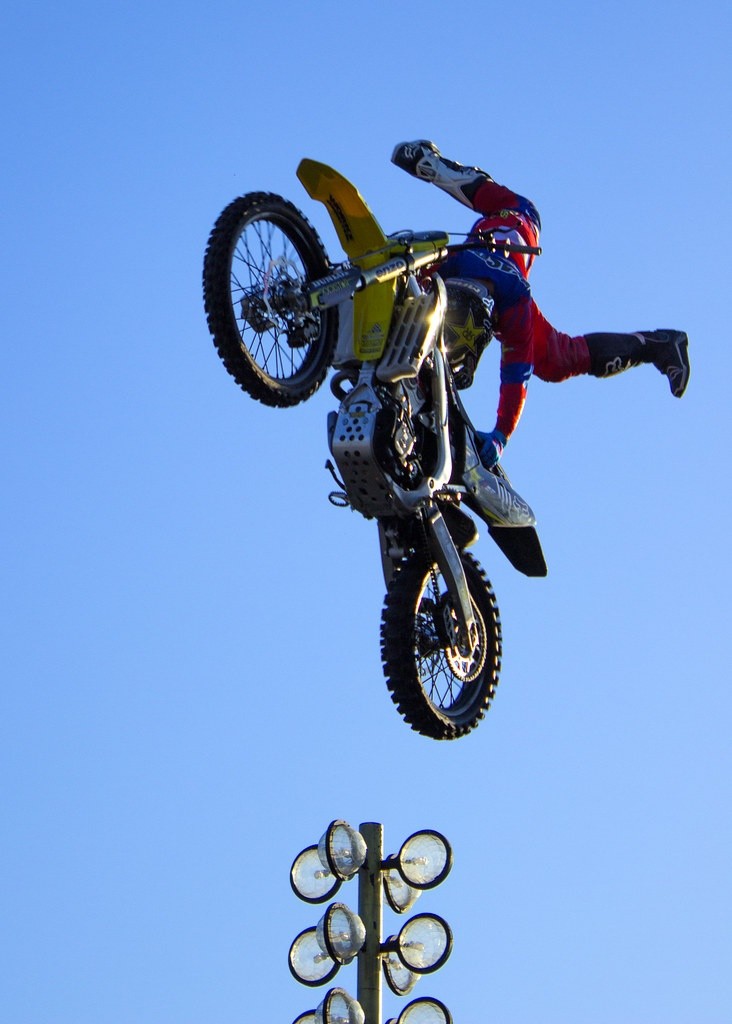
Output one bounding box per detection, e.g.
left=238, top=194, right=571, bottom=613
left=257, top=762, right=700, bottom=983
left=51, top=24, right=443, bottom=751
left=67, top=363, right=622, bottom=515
left=390, top=140, right=688, bottom=473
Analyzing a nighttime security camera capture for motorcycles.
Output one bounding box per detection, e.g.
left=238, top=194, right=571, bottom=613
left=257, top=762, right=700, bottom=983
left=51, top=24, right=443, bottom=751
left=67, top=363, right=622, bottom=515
left=200, top=158, right=547, bottom=741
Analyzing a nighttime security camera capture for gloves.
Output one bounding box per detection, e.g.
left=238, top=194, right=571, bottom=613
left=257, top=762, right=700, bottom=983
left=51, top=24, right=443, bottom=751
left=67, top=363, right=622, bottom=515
left=475, top=428, right=507, bottom=473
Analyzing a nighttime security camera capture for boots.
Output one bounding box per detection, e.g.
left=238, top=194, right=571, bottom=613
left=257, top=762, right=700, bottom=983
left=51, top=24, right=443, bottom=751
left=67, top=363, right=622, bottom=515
left=582, top=329, right=690, bottom=397
left=391, top=140, right=493, bottom=212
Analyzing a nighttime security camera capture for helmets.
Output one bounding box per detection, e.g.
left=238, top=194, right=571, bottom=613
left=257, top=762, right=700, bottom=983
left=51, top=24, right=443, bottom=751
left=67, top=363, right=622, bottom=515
left=438, top=276, right=493, bottom=389
